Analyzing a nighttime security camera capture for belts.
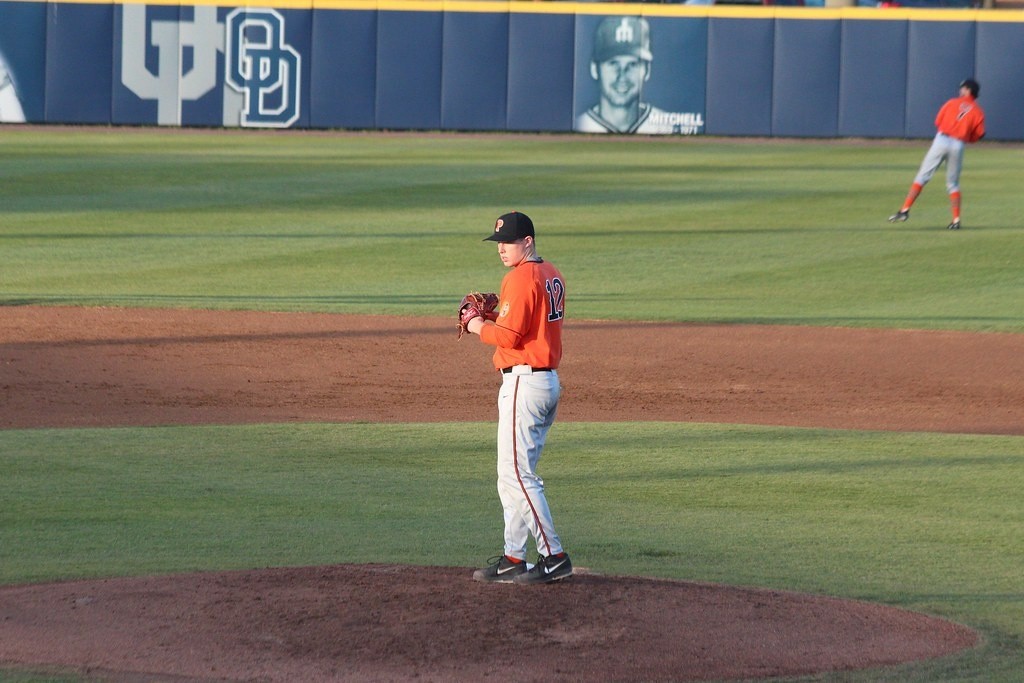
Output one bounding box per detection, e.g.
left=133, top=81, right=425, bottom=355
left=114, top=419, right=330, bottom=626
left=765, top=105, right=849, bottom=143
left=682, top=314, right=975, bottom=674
left=498, top=365, right=553, bottom=374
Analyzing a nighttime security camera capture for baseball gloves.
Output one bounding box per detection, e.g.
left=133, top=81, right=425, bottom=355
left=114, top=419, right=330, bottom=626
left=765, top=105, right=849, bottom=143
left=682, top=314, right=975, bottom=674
left=457, top=292, right=500, bottom=339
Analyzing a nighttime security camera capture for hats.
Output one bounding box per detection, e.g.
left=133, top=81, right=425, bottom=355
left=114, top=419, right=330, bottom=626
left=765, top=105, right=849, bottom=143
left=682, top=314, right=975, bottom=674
left=590, top=15, right=653, bottom=63
left=958, top=79, right=980, bottom=90
left=482, top=210, right=535, bottom=244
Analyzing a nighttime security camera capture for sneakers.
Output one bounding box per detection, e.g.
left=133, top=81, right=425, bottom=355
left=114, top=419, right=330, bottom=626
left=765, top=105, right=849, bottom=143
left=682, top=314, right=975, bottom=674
left=471, top=556, right=527, bottom=584
left=514, top=552, right=574, bottom=587
left=946, top=221, right=961, bottom=230
left=888, top=209, right=908, bottom=223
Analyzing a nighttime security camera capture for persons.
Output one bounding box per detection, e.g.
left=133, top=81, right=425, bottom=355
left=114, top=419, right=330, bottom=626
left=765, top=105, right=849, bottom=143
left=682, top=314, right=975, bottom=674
left=575, top=16, right=687, bottom=135
left=888, top=76, right=984, bottom=231
left=457, top=210, right=573, bottom=585
